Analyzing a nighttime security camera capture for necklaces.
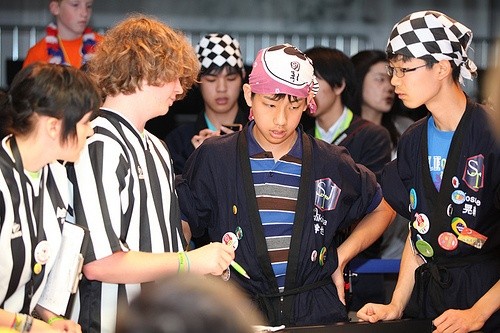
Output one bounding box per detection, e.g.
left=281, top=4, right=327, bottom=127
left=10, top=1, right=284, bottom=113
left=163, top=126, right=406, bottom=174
left=46, top=22, right=97, bottom=67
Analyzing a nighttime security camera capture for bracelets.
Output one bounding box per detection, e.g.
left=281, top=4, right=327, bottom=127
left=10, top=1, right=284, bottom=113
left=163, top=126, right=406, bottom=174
left=47, top=316, right=63, bottom=325
left=183, top=252, right=191, bottom=273
left=10, top=311, right=22, bottom=331
left=177, top=252, right=184, bottom=272
left=21, top=314, right=33, bottom=333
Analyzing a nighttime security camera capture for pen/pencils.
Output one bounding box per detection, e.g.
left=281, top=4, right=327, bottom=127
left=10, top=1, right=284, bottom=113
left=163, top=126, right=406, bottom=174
left=210, top=241, right=250, bottom=278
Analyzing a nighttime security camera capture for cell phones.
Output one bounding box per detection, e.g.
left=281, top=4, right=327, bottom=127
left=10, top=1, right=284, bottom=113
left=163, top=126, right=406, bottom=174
left=221, top=124, right=241, bottom=135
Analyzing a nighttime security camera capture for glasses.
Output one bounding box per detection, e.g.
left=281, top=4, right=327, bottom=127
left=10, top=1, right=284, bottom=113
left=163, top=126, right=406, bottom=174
left=385, top=64, right=429, bottom=78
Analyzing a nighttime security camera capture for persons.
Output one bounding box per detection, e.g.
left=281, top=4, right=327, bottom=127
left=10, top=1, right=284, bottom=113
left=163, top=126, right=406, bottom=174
left=350, top=49, right=415, bottom=261
left=22, top=0, right=107, bottom=69
left=61, top=10, right=235, bottom=333
left=164, top=31, right=251, bottom=176
left=298, top=46, right=392, bottom=313
left=179, top=42, right=397, bottom=328
left=356, top=10, right=500, bottom=333
left=0, top=61, right=101, bottom=333
